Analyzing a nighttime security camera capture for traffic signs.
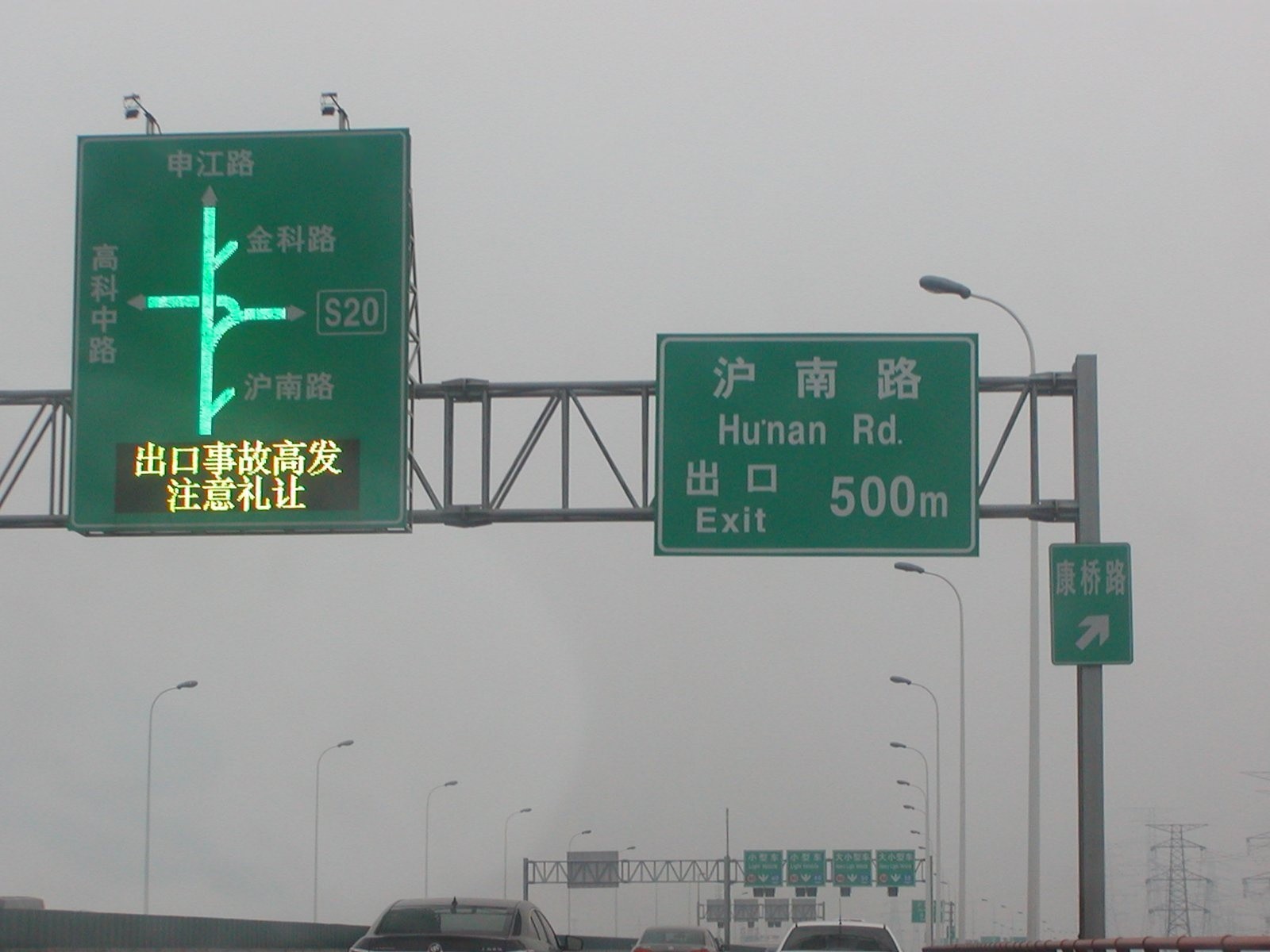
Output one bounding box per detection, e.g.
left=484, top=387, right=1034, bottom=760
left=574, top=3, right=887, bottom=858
left=831, top=849, right=873, bottom=887
left=875, top=849, right=917, bottom=887
left=743, top=850, right=784, bottom=888
left=784, top=850, right=828, bottom=886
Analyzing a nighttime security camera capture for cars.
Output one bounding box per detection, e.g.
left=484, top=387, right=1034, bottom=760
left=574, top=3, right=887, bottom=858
left=630, top=925, right=726, bottom=952
left=348, top=896, right=585, bottom=952
left=765, top=919, right=906, bottom=952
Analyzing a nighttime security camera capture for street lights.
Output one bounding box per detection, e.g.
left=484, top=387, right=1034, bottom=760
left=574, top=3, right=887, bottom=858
left=144, top=679, right=201, bottom=914
left=424, top=781, right=459, bottom=897
left=614, top=846, right=637, bottom=937
left=919, top=274, right=1044, bottom=941
left=503, top=807, right=533, bottom=897
left=313, top=740, right=355, bottom=927
left=893, top=561, right=967, bottom=941
left=891, top=675, right=941, bottom=946
left=566, top=830, right=592, bottom=935
